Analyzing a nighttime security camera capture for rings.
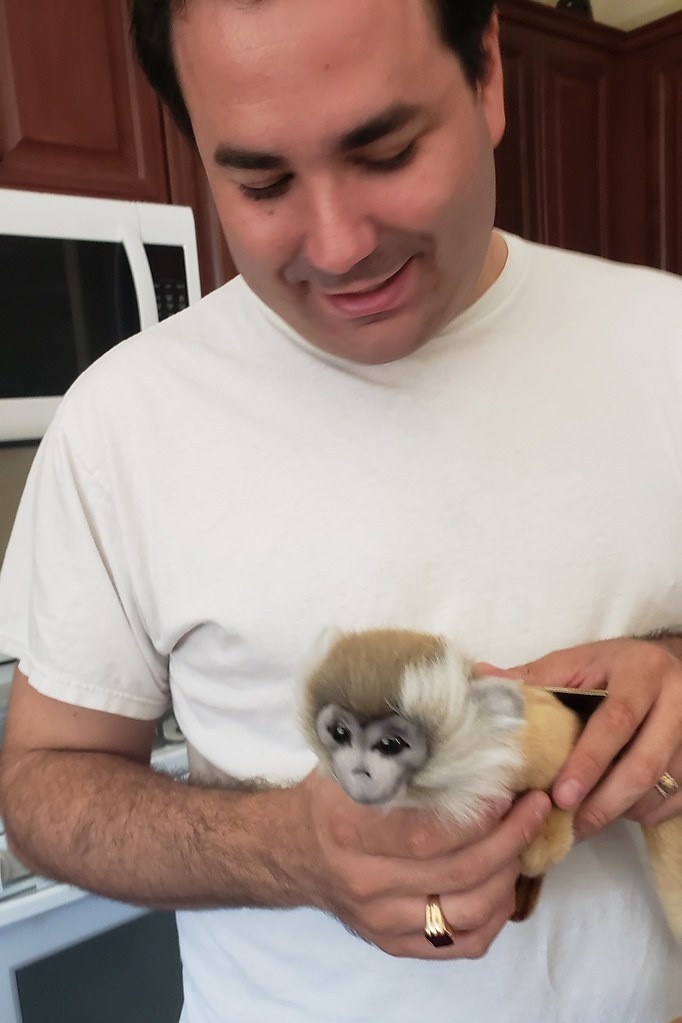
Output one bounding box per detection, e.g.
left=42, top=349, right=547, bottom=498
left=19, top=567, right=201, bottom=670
left=424, top=894, right=456, bottom=948
left=655, top=771, right=680, bottom=798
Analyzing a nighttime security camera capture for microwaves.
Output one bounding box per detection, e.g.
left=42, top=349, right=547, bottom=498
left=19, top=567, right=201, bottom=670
left=1, top=185, right=201, bottom=447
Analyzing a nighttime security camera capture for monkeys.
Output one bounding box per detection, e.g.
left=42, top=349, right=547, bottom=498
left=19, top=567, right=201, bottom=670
left=289, top=621, right=682, bottom=951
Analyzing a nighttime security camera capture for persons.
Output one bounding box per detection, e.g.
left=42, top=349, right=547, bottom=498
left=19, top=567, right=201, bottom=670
left=0, top=0, right=682, bottom=1023
